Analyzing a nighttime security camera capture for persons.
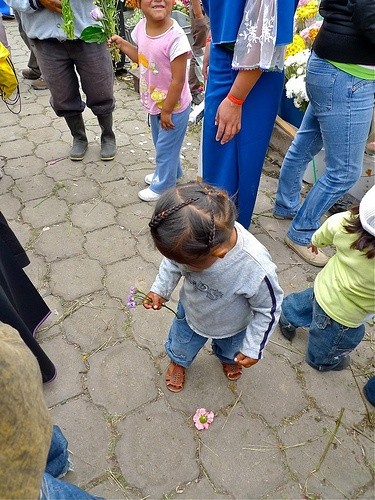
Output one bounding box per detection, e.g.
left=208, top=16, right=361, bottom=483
left=276, top=184, right=375, bottom=371
left=200, top=0, right=299, bottom=230
left=106, top=0, right=193, bottom=202
left=0, top=320, right=104, bottom=500
left=273, top=1, right=375, bottom=267
left=142, top=180, right=284, bottom=392
left=189, top=0, right=212, bottom=105
left=0, top=211, right=57, bottom=384
left=0, top=0, right=118, bottom=161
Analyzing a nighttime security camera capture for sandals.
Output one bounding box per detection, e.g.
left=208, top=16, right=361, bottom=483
left=166, top=359, right=185, bottom=393
left=223, top=360, right=241, bottom=381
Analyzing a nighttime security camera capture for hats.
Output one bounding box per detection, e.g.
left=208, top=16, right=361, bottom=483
left=358, top=184, right=375, bottom=237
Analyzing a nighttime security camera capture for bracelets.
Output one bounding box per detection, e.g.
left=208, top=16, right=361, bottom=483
left=226, top=94, right=244, bottom=105
left=206, top=37, right=211, bottom=43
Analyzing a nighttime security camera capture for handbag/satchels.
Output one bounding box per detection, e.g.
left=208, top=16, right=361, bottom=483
left=0, top=42, right=19, bottom=100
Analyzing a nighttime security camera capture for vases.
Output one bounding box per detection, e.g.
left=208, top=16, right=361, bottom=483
left=123, top=24, right=141, bottom=46
left=279, top=92, right=313, bottom=129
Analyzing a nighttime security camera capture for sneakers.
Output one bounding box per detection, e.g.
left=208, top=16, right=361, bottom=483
left=138, top=187, right=162, bottom=202
left=286, top=235, right=331, bottom=267
left=273, top=214, right=294, bottom=220
left=145, top=173, right=154, bottom=185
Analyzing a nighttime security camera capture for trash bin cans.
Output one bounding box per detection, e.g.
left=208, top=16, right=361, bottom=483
left=124, top=10, right=196, bottom=83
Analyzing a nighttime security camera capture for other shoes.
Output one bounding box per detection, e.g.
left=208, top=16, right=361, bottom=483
left=21, top=68, right=40, bottom=78
left=31, top=76, right=48, bottom=90
left=334, top=353, right=351, bottom=370
left=280, top=321, right=296, bottom=341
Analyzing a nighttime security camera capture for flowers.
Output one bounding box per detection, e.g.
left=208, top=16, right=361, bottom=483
left=285, top=0, right=323, bottom=112
left=127, top=286, right=178, bottom=321
left=76, top=0, right=150, bottom=61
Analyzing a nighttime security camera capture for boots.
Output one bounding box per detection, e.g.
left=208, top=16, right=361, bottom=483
left=65, top=115, right=87, bottom=160
left=96, top=113, right=116, bottom=161
